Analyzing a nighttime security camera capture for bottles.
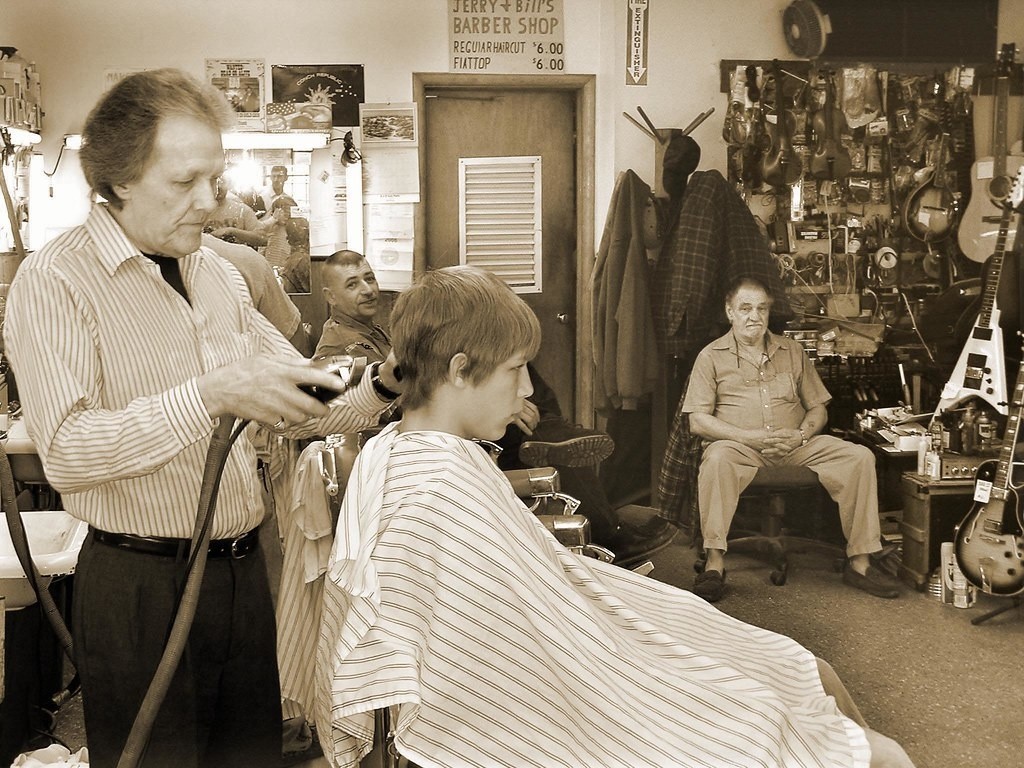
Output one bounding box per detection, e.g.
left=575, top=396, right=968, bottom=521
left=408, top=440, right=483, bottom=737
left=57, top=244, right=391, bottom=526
left=941, top=542, right=954, bottom=605
left=917, top=415, right=944, bottom=481
left=975, top=411, right=992, bottom=457
left=960, top=406, right=975, bottom=457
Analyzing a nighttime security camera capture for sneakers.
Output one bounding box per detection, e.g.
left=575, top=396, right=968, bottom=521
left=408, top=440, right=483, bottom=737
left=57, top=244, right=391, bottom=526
left=519, top=414, right=615, bottom=468
left=598, top=521, right=678, bottom=566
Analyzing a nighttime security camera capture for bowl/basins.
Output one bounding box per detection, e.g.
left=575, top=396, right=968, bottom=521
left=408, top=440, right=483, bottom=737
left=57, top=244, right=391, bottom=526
left=0, top=511, right=89, bottom=611
left=0, top=414, right=47, bottom=484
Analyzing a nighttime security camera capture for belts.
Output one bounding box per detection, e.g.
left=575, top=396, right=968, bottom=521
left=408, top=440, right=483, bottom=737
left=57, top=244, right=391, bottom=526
left=93, top=529, right=258, bottom=558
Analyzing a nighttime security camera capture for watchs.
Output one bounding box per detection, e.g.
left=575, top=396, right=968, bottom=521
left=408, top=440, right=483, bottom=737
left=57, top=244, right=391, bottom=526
left=799, top=428, right=808, bottom=446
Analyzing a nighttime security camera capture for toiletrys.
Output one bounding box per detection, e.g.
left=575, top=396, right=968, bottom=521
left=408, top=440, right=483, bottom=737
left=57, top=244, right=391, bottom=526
left=917, top=431, right=941, bottom=481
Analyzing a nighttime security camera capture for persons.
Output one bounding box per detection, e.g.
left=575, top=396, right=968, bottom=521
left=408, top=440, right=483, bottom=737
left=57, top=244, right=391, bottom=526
left=201, top=232, right=307, bottom=355
left=681, top=276, right=900, bottom=603
left=354, top=265, right=914, bottom=768
left=310, top=249, right=679, bottom=577
left=202, top=165, right=309, bottom=293
left=2, top=70, right=405, bottom=768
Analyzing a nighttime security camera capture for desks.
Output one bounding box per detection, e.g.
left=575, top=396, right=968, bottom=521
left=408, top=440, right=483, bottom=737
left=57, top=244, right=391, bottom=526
left=899, top=473, right=975, bottom=589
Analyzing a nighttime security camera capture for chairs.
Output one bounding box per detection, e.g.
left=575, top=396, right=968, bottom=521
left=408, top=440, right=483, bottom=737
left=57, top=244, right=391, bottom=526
left=694, top=465, right=845, bottom=585
left=312, top=433, right=653, bottom=768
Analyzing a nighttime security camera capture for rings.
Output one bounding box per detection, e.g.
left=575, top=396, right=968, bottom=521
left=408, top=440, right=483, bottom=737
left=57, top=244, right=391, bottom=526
left=273, top=416, right=285, bottom=428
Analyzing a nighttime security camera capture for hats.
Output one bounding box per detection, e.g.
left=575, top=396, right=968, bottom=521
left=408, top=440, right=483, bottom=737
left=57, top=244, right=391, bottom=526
left=663, top=135, right=702, bottom=198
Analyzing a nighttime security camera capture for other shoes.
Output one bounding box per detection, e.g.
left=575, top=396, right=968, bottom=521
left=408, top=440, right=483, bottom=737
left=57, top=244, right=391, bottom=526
left=844, top=564, right=899, bottom=598
left=283, top=727, right=324, bottom=765
left=693, top=570, right=730, bottom=602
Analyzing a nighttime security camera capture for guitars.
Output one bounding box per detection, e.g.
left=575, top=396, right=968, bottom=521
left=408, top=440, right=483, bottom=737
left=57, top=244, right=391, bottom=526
left=954, top=44, right=1023, bottom=264
left=901, top=64, right=960, bottom=244
left=953, top=329, right=1024, bottom=599
left=926, top=165, right=1023, bottom=430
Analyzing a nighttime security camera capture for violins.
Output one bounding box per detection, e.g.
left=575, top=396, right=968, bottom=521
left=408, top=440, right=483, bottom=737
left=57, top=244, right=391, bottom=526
left=808, top=65, right=852, bottom=183
left=752, top=60, right=805, bottom=188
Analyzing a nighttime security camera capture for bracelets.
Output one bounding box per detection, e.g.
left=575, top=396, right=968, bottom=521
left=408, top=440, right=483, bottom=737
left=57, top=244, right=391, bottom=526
left=372, top=362, right=402, bottom=400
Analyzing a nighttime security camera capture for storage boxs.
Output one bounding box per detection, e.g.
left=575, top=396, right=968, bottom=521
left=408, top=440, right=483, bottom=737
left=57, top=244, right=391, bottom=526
left=818, top=325, right=841, bottom=355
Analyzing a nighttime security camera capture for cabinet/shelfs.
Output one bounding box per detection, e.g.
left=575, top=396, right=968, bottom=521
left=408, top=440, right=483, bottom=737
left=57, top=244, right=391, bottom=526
left=783, top=330, right=819, bottom=363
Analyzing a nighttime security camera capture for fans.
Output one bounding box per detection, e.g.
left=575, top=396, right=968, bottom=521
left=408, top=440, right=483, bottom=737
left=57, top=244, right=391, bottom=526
left=782, top=1, right=832, bottom=60
